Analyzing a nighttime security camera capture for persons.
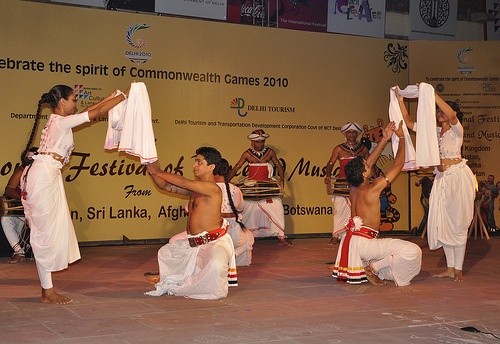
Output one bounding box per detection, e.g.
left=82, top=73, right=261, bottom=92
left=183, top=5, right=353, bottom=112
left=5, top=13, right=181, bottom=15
left=184, top=158, right=255, bottom=266
left=323, top=121, right=370, bottom=240
left=226, top=129, right=296, bottom=248
left=332, top=120, right=423, bottom=288
left=0, top=146, right=41, bottom=264
left=391, top=82, right=479, bottom=282
left=20, top=84, right=138, bottom=305
left=142, top=146, right=238, bottom=300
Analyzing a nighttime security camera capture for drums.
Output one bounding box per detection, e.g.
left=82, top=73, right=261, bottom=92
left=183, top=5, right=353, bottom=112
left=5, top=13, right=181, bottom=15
left=324, top=174, right=351, bottom=197
left=0, top=192, right=31, bottom=220
left=233, top=180, right=286, bottom=201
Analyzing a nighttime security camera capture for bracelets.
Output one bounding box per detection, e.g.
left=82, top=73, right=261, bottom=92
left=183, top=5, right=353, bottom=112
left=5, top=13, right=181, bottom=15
left=399, top=136, right=406, bottom=139
left=280, top=179, right=284, bottom=181
left=120, top=91, right=127, bottom=100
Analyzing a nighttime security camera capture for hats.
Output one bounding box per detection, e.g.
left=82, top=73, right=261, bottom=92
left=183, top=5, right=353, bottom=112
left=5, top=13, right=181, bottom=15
left=247, top=129, right=270, bottom=141
left=25, top=147, right=40, bottom=158
left=341, top=121, right=362, bottom=133
